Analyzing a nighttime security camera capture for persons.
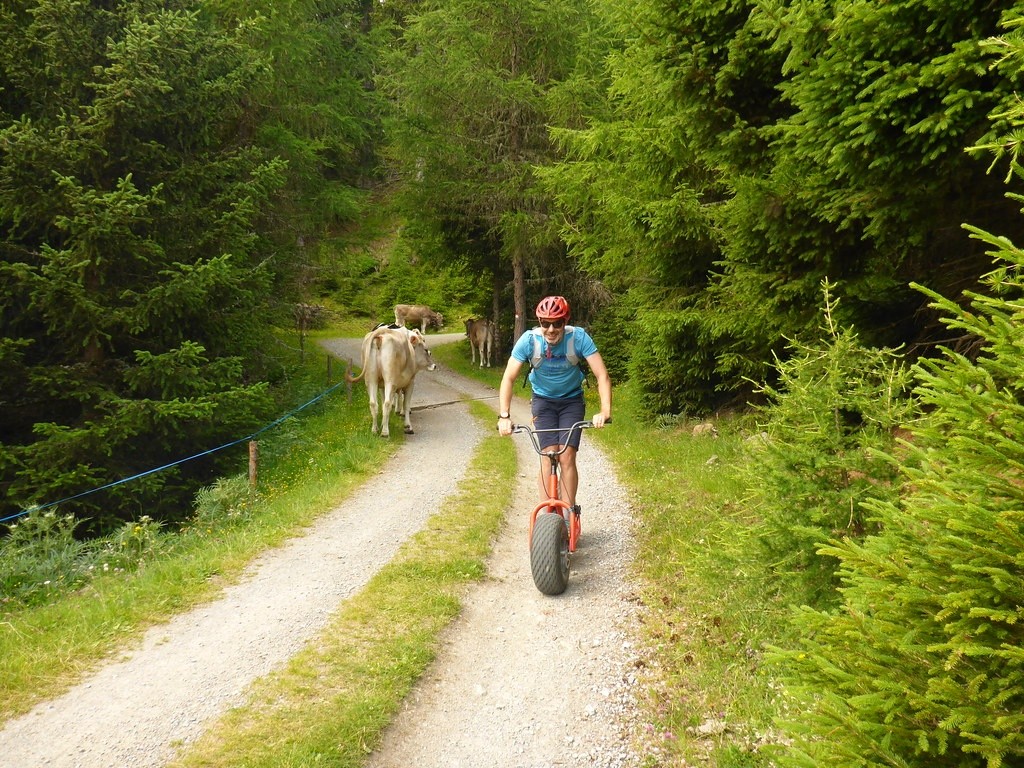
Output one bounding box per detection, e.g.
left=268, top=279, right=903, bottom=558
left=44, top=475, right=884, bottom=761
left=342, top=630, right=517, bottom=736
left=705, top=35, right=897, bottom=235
left=498, top=295, right=612, bottom=535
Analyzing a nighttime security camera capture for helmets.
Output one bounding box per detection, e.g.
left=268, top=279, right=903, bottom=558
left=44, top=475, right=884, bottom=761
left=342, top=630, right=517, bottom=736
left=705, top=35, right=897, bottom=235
left=536, top=295, right=570, bottom=323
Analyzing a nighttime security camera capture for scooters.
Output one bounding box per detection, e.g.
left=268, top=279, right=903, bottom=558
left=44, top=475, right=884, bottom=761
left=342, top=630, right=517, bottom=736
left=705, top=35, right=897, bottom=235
left=495, top=418, right=613, bottom=597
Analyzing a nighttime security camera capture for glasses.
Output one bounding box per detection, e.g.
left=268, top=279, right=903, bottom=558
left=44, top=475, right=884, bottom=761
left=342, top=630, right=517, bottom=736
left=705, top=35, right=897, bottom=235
left=540, top=319, right=564, bottom=328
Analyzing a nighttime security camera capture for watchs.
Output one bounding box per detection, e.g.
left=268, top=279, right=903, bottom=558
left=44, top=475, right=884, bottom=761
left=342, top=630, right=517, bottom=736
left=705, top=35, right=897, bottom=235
left=498, top=412, right=510, bottom=419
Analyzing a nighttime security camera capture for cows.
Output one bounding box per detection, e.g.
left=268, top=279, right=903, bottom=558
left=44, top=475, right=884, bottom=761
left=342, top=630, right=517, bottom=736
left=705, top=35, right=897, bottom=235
left=392, top=304, right=445, bottom=335
left=463, top=318, right=495, bottom=368
left=343, top=320, right=436, bottom=437
